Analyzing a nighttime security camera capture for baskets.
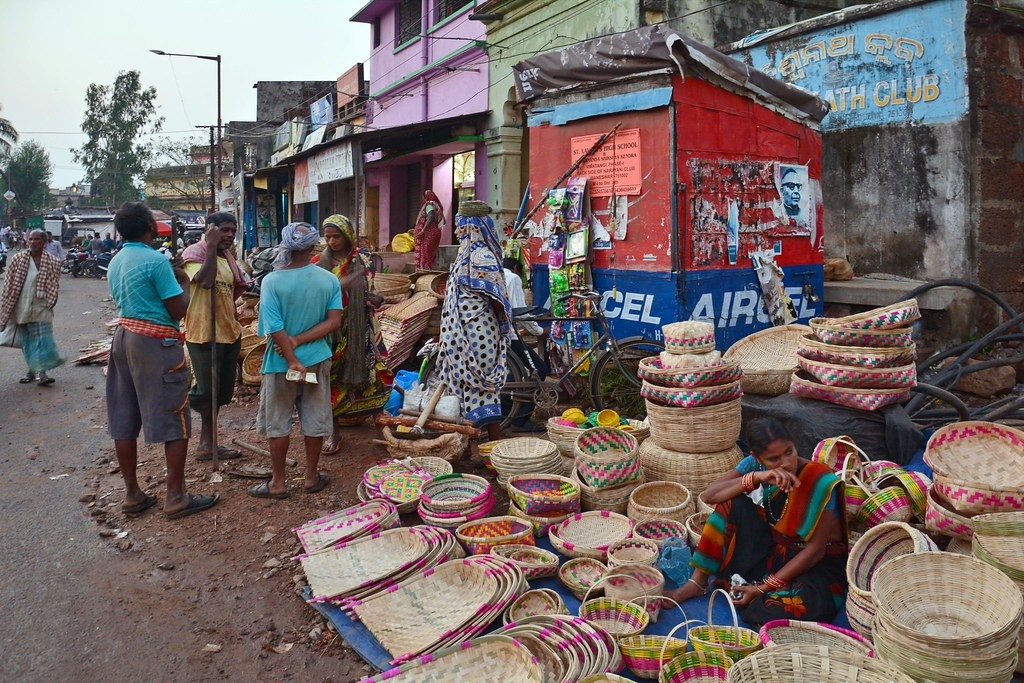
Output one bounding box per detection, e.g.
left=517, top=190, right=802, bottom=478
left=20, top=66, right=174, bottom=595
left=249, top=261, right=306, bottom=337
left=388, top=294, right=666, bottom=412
left=357, top=272, right=1024, bottom=683
left=242, top=343, right=268, bottom=385
left=240, top=333, right=267, bottom=358
left=239, top=316, right=258, bottom=329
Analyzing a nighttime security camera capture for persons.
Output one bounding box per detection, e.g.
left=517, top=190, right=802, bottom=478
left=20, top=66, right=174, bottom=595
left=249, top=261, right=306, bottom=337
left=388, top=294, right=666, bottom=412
left=499, top=257, right=553, bottom=432
left=44, top=231, right=62, bottom=261
left=408, top=190, right=445, bottom=272
left=1, top=223, right=35, bottom=260
left=247, top=221, right=344, bottom=499
left=181, top=211, right=247, bottom=461
left=309, top=214, right=388, bottom=455
left=438, top=200, right=510, bottom=478
left=72, top=230, right=199, bottom=259
left=1, top=229, right=64, bottom=385
left=780, top=165, right=806, bottom=225
left=661, top=415, right=849, bottom=629
left=104, top=200, right=220, bottom=519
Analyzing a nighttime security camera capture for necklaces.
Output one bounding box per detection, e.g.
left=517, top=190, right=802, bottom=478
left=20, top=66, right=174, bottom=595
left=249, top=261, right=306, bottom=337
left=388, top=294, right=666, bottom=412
left=767, top=483, right=790, bottom=522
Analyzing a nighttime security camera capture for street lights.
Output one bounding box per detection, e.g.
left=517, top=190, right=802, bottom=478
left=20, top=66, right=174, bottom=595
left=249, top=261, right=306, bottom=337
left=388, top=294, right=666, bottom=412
left=2, top=161, right=10, bottom=226
left=148, top=49, right=224, bottom=213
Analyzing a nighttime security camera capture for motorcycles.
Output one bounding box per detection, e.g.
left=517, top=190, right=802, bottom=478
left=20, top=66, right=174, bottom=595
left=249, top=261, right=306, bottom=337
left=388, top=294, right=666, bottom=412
left=60, top=223, right=205, bottom=280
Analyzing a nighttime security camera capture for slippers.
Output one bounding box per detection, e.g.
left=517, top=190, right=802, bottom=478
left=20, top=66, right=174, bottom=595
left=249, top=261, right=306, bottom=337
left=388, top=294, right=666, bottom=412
left=247, top=480, right=289, bottom=498
left=122, top=495, right=157, bottom=513
left=304, top=475, right=330, bottom=493
left=166, top=493, right=220, bottom=519
left=196, top=446, right=241, bottom=461
left=321, top=436, right=344, bottom=454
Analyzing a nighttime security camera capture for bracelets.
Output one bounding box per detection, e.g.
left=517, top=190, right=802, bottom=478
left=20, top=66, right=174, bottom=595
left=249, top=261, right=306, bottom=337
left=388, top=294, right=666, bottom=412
left=742, top=469, right=760, bottom=492
left=753, top=573, right=787, bottom=595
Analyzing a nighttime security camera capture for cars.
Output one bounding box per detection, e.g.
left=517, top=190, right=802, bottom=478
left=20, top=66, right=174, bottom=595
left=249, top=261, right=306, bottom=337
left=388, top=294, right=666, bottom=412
left=0, top=242, right=10, bottom=270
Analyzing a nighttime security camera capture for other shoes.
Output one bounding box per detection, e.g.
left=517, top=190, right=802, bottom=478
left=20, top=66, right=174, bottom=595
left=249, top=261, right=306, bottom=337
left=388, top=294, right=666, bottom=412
left=37, top=376, right=55, bottom=385
left=20, top=372, right=34, bottom=383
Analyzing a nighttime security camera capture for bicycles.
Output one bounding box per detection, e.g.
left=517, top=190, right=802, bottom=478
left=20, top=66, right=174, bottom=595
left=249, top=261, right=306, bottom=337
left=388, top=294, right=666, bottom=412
left=415, top=291, right=667, bottom=442
left=8, top=234, right=22, bottom=251
